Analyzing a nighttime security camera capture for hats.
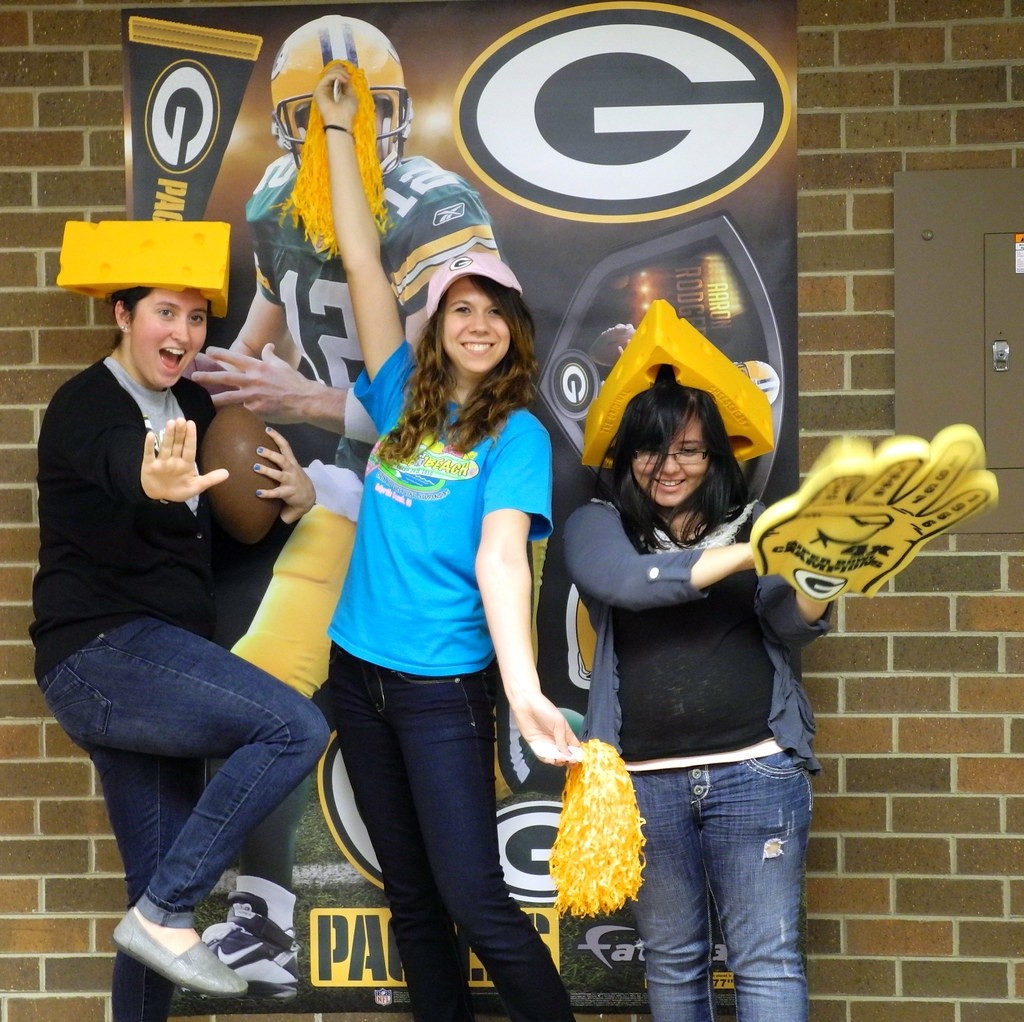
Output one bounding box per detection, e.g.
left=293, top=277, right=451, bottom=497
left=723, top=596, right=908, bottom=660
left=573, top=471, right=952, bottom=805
left=424, top=251, right=523, bottom=320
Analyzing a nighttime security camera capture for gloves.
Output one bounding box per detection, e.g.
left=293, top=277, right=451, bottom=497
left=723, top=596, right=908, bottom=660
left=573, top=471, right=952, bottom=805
left=750, top=424, right=998, bottom=604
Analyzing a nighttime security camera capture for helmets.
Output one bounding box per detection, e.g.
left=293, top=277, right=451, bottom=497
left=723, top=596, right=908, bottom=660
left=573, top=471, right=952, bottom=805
left=269, top=14, right=405, bottom=165
left=734, top=359, right=780, bottom=406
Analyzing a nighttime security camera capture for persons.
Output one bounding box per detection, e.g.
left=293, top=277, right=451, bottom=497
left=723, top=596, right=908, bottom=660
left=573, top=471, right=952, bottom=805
left=323, top=60, right=583, bottom=1022
left=179, top=7, right=549, bottom=1002
left=37, top=274, right=330, bottom=1022
left=560, top=363, right=851, bottom=1022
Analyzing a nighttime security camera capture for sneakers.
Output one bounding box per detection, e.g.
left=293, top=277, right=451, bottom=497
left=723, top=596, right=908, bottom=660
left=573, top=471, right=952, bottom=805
left=181, top=892, right=299, bottom=1001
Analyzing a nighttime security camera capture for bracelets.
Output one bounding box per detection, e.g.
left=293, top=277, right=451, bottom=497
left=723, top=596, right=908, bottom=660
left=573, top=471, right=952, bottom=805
left=324, top=125, right=356, bottom=145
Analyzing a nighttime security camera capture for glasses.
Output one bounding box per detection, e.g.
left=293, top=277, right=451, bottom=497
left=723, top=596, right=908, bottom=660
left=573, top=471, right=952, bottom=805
left=636, top=447, right=709, bottom=465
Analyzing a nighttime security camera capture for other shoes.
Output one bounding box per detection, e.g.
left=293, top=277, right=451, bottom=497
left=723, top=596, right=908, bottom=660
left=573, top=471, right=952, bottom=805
left=110, top=906, right=247, bottom=997
